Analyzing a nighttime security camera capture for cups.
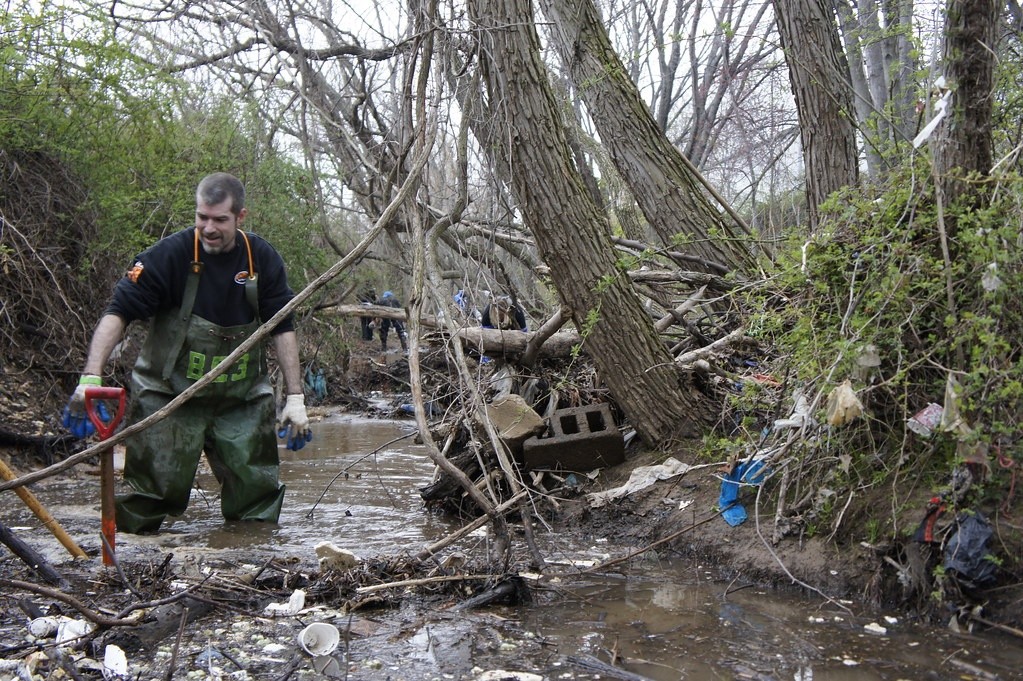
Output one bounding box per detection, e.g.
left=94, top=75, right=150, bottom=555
left=296, top=622, right=340, bottom=657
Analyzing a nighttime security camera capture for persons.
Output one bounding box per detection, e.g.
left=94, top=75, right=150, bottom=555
left=357, top=279, right=375, bottom=340
left=372, top=291, right=407, bottom=351
left=60, top=171, right=312, bottom=535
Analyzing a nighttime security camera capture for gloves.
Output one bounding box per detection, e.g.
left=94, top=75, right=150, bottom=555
left=62, top=373, right=111, bottom=438
left=280, top=393, right=313, bottom=452
